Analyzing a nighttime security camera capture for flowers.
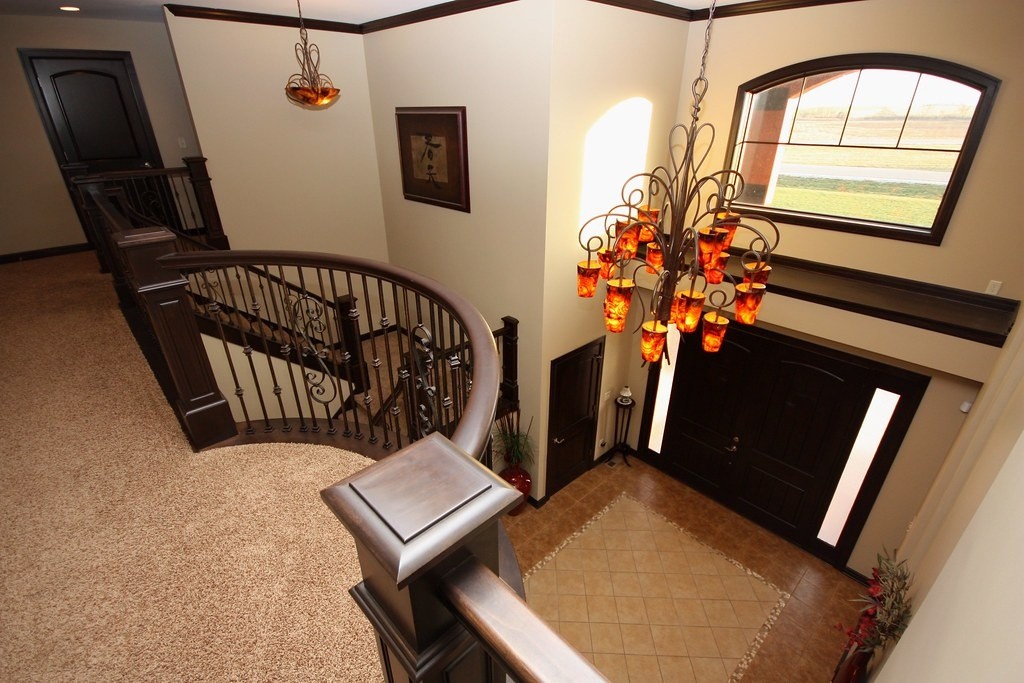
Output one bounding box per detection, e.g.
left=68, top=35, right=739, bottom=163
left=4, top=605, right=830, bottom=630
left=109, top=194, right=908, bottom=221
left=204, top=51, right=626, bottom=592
left=830, top=544, right=913, bottom=661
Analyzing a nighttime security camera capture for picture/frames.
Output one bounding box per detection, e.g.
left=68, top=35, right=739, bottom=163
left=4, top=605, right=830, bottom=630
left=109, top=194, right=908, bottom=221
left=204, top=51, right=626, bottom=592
left=395, top=105, right=471, bottom=213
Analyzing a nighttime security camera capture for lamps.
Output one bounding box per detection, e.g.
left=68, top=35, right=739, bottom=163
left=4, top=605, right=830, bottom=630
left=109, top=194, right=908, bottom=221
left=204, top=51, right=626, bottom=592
left=620, top=386, right=632, bottom=403
left=284, top=0, right=340, bottom=107
left=576, top=0, right=780, bottom=367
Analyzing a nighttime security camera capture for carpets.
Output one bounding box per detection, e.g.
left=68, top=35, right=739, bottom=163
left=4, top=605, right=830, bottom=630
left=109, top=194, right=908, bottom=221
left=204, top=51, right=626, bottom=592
left=522, top=491, right=790, bottom=683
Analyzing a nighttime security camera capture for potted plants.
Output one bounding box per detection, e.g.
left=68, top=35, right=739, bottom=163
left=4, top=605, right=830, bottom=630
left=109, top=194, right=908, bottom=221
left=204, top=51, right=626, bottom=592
left=491, top=429, right=537, bottom=517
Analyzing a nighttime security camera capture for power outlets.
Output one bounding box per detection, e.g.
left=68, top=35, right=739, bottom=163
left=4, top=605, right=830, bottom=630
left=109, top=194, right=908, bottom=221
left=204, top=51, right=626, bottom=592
left=985, top=280, right=1003, bottom=295
